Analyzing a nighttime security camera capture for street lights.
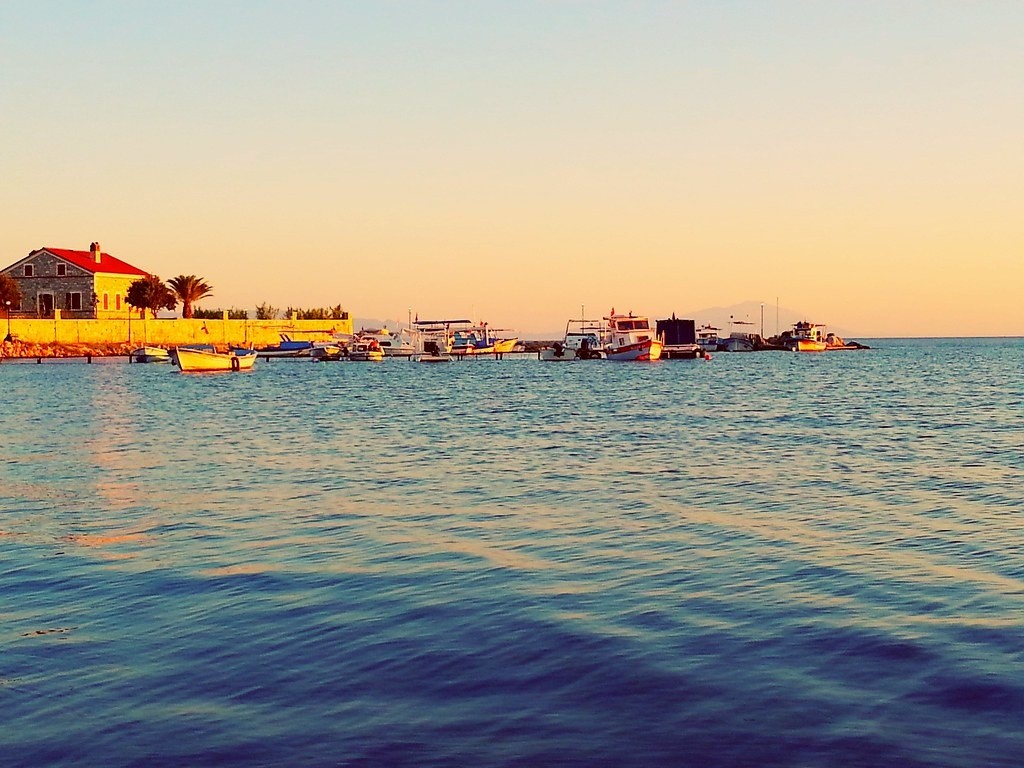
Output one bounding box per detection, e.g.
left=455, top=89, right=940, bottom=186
left=128, top=303, right=133, bottom=344
left=5, top=300, right=14, bottom=342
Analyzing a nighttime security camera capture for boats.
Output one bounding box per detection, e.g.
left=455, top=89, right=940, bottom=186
left=356, top=307, right=720, bottom=363
left=308, top=345, right=341, bottom=362
left=131, top=346, right=169, bottom=363
left=722, top=333, right=755, bottom=352
left=349, top=340, right=384, bottom=362
left=167, top=340, right=259, bottom=372
left=784, top=320, right=828, bottom=352
